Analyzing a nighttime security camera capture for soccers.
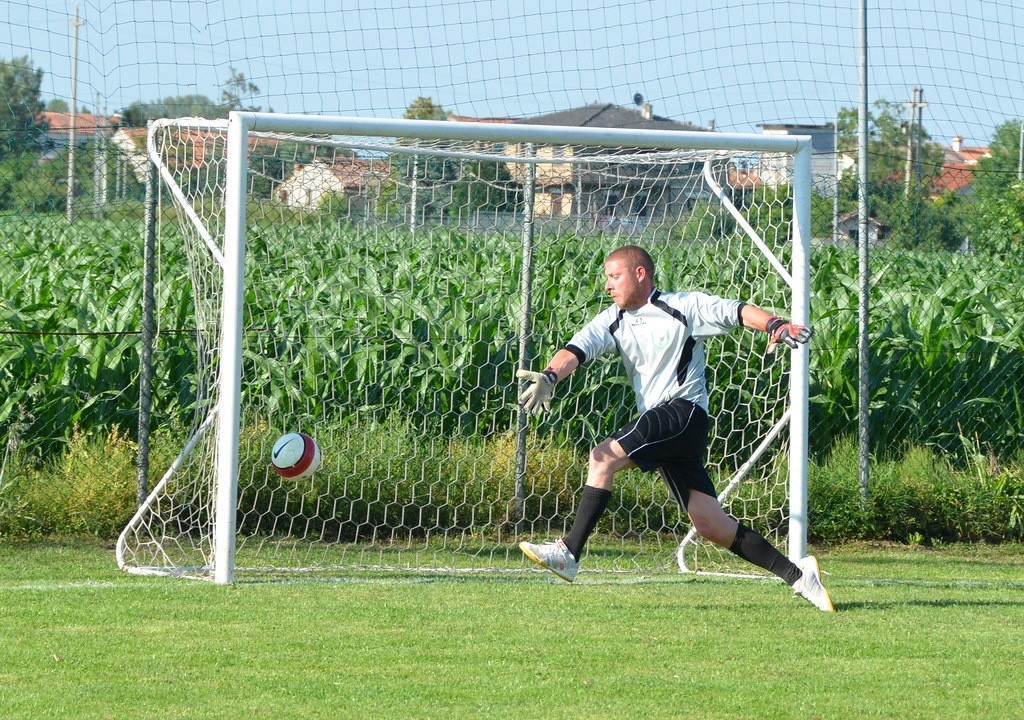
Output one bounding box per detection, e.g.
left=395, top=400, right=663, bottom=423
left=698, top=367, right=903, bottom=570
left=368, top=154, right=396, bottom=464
left=270, top=433, right=322, bottom=481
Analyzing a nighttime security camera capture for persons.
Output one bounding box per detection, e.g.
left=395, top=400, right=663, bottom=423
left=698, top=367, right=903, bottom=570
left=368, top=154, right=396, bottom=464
left=514, top=245, right=835, bottom=613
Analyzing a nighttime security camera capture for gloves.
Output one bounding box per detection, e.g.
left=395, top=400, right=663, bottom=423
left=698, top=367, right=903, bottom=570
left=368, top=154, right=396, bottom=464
left=764, top=316, right=811, bottom=355
left=516, top=368, right=557, bottom=415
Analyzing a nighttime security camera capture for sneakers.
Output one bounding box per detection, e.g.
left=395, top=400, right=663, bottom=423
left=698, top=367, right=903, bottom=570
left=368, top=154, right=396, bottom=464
left=519, top=538, right=580, bottom=583
left=792, top=555, right=833, bottom=612
